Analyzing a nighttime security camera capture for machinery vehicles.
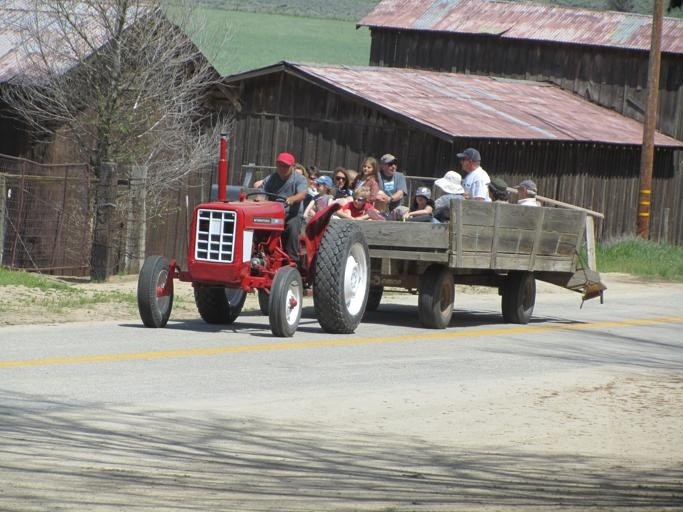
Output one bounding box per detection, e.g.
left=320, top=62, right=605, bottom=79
left=137, top=129, right=374, bottom=338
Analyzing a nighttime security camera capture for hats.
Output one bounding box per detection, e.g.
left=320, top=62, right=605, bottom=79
left=485, top=179, right=506, bottom=192
left=513, top=179, right=537, bottom=192
left=276, top=152, right=295, bottom=166
left=416, top=187, right=432, bottom=200
left=316, top=176, right=332, bottom=187
left=435, top=171, right=465, bottom=195
left=380, top=154, right=397, bottom=164
left=456, top=148, right=481, bottom=161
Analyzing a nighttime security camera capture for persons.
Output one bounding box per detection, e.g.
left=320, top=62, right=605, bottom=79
left=251, top=148, right=542, bottom=264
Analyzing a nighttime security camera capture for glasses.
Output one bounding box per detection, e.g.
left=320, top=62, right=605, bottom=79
left=334, top=176, right=346, bottom=181
left=385, top=160, right=397, bottom=166
